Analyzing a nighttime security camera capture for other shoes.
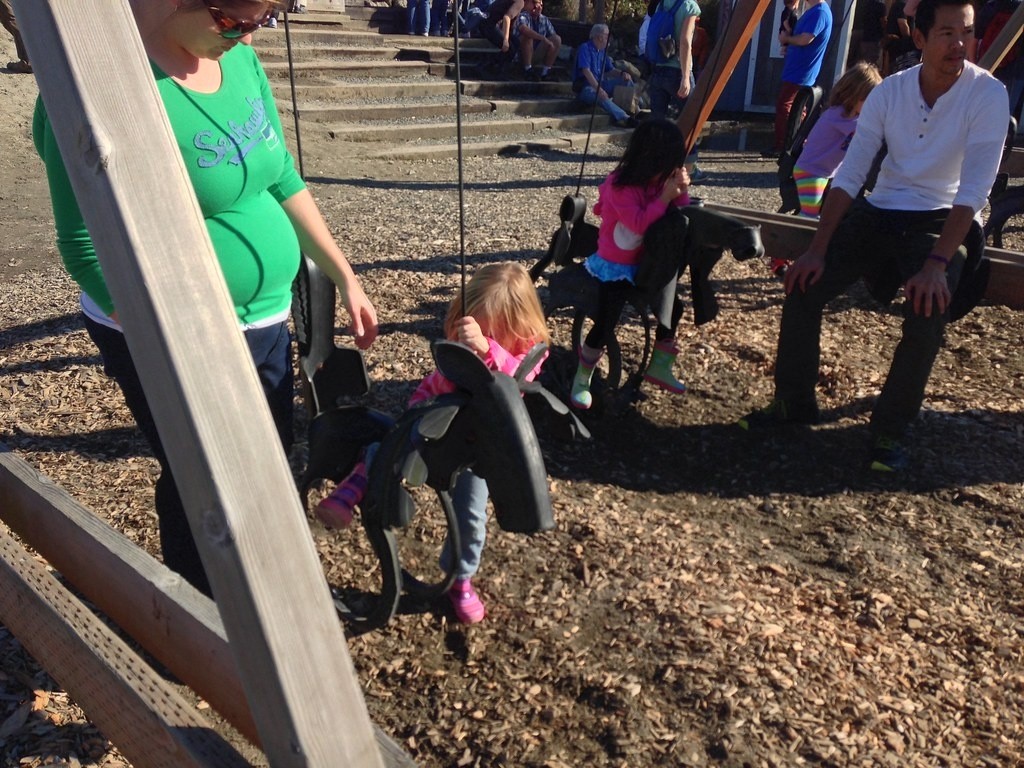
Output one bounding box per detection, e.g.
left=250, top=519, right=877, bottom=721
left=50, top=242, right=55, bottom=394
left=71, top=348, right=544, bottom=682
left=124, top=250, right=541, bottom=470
left=422, top=32, right=428, bottom=36
left=313, top=464, right=369, bottom=530
left=408, top=32, right=416, bottom=34
left=431, top=30, right=447, bottom=36
left=686, top=165, right=709, bottom=183
left=459, top=32, right=470, bottom=37
left=611, top=117, right=640, bottom=129
left=760, top=143, right=785, bottom=157
left=524, top=68, right=539, bottom=81
left=541, top=69, right=559, bottom=82
left=441, top=574, right=485, bottom=624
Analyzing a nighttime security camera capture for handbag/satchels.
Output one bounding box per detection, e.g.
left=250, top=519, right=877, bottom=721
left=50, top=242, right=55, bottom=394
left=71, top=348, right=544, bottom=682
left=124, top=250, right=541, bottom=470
left=613, top=79, right=650, bottom=116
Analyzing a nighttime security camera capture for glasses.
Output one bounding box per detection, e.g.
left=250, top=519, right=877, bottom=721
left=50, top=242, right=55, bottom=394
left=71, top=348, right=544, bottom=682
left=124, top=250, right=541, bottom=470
left=203, top=0, right=260, bottom=40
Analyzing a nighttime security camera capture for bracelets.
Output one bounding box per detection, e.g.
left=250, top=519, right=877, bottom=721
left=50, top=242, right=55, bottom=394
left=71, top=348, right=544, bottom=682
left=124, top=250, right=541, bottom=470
left=927, top=253, right=949, bottom=264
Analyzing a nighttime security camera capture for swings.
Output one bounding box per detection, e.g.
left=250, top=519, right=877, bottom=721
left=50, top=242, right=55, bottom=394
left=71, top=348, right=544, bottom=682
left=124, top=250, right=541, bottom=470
left=528, top=0, right=767, bottom=328
left=772, top=1, right=857, bottom=218
left=276, top=0, right=594, bottom=536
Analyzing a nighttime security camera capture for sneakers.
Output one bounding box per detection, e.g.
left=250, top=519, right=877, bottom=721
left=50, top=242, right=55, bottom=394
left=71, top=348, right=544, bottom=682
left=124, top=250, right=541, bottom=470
left=869, top=431, right=900, bottom=472
left=738, top=393, right=819, bottom=431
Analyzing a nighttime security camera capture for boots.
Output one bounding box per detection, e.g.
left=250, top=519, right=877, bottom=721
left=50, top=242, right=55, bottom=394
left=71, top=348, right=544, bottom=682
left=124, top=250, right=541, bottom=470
left=570, top=353, right=598, bottom=409
left=642, top=340, right=685, bottom=395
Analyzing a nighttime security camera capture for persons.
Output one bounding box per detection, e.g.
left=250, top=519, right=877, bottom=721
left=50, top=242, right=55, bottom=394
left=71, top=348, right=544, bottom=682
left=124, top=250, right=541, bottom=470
left=644, top=0, right=711, bottom=182
left=778, top=0, right=800, bottom=56
left=569, top=116, right=694, bottom=410
left=571, top=23, right=651, bottom=127
left=776, top=0, right=833, bottom=215
left=313, top=260, right=557, bottom=624
left=404, top=0, right=562, bottom=83
left=32, top=0, right=381, bottom=629
left=738, top=0, right=1011, bottom=476
left=793, top=59, right=888, bottom=221
left=638, top=0, right=659, bottom=59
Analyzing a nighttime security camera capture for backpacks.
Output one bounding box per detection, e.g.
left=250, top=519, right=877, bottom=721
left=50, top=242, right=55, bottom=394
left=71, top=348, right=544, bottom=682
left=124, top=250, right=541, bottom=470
left=645, top=0, right=685, bottom=63
